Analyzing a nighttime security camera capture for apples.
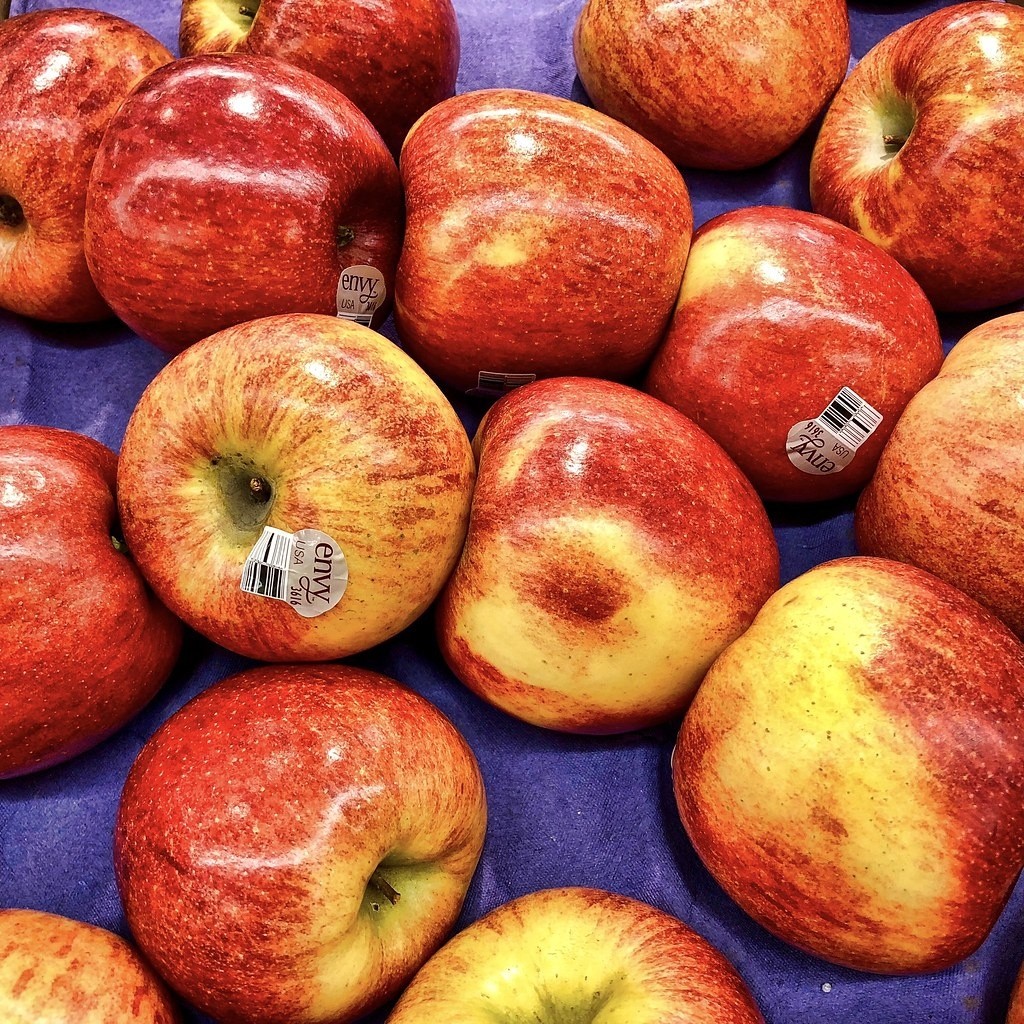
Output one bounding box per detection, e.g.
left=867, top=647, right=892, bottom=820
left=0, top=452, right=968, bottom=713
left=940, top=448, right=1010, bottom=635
left=0, top=0, right=1024, bottom=1024
